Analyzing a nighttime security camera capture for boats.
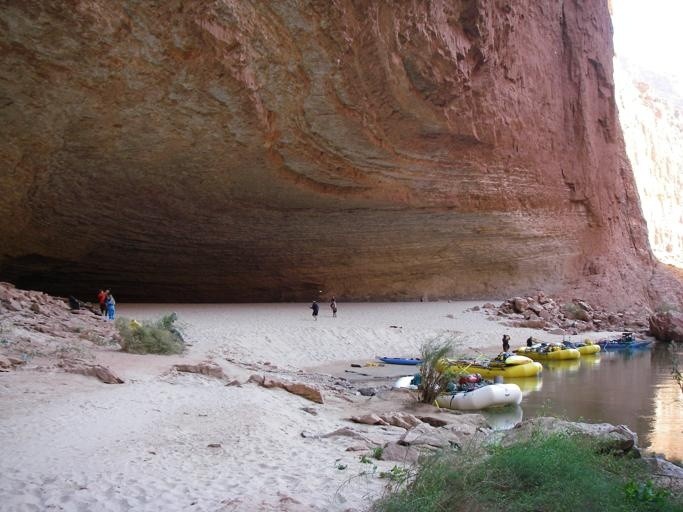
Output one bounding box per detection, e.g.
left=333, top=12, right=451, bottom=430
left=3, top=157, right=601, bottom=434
left=478, top=405, right=523, bottom=434
left=395, top=373, right=521, bottom=411
left=436, top=353, right=543, bottom=377
left=572, top=339, right=600, bottom=355
left=579, top=353, right=601, bottom=366
left=536, top=357, right=580, bottom=372
left=375, top=355, right=426, bottom=365
left=517, top=343, right=580, bottom=361
left=597, top=330, right=651, bottom=347
left=601, top=344, right=653, bottom=353
left=498, top=374, right=542, bottom=396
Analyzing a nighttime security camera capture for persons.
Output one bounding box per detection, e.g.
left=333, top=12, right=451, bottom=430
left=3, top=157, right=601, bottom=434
left=330, top=295, right=335, bottom=305
left=308, top=301, right=319, bottom=320
left=105, top=293, right=116, bottom=320
left=96, top=288, right=106, bottom=316
left=527, top=336, right=532, bottom=347
left=329, top=299, right=336, bottom=318
left=502, top=334, right=511, bottom=352
left=104, top=286, right=111, bottom=295
